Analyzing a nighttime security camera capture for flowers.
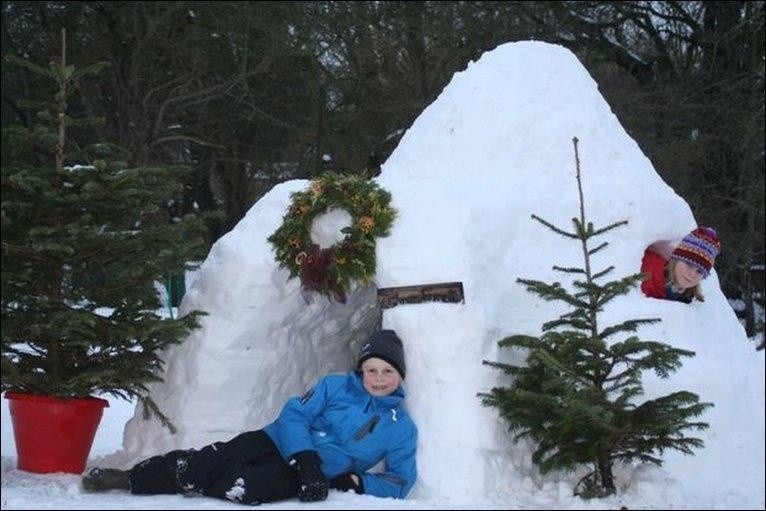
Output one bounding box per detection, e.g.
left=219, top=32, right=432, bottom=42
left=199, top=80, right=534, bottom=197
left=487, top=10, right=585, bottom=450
left=267, top=169, right=403, bottom=306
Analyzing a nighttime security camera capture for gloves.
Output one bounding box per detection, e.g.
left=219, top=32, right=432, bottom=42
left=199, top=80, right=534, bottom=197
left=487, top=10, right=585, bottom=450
left=296, top=462, right=328, bottom=502
left=329, top=473, right=357, bottom=491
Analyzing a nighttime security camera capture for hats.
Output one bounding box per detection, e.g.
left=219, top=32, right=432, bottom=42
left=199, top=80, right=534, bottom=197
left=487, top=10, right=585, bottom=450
left=356, top=329, right=405, bottom=382
left=672, top=226, right=722, bottom=280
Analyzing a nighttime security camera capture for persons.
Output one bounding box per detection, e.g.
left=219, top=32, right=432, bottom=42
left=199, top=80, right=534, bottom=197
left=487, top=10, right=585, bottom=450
left=82, top=329, right=417, bottom=506
left=641, top=227, right=722, bottom=304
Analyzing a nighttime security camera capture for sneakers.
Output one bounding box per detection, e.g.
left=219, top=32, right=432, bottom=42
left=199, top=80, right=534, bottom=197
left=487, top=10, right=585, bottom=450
left=79, top=466, right=128, bottom=493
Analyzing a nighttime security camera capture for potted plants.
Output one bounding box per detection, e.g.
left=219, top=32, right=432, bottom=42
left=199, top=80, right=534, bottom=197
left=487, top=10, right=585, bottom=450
left=0, top=24, right=228, bottom=474
